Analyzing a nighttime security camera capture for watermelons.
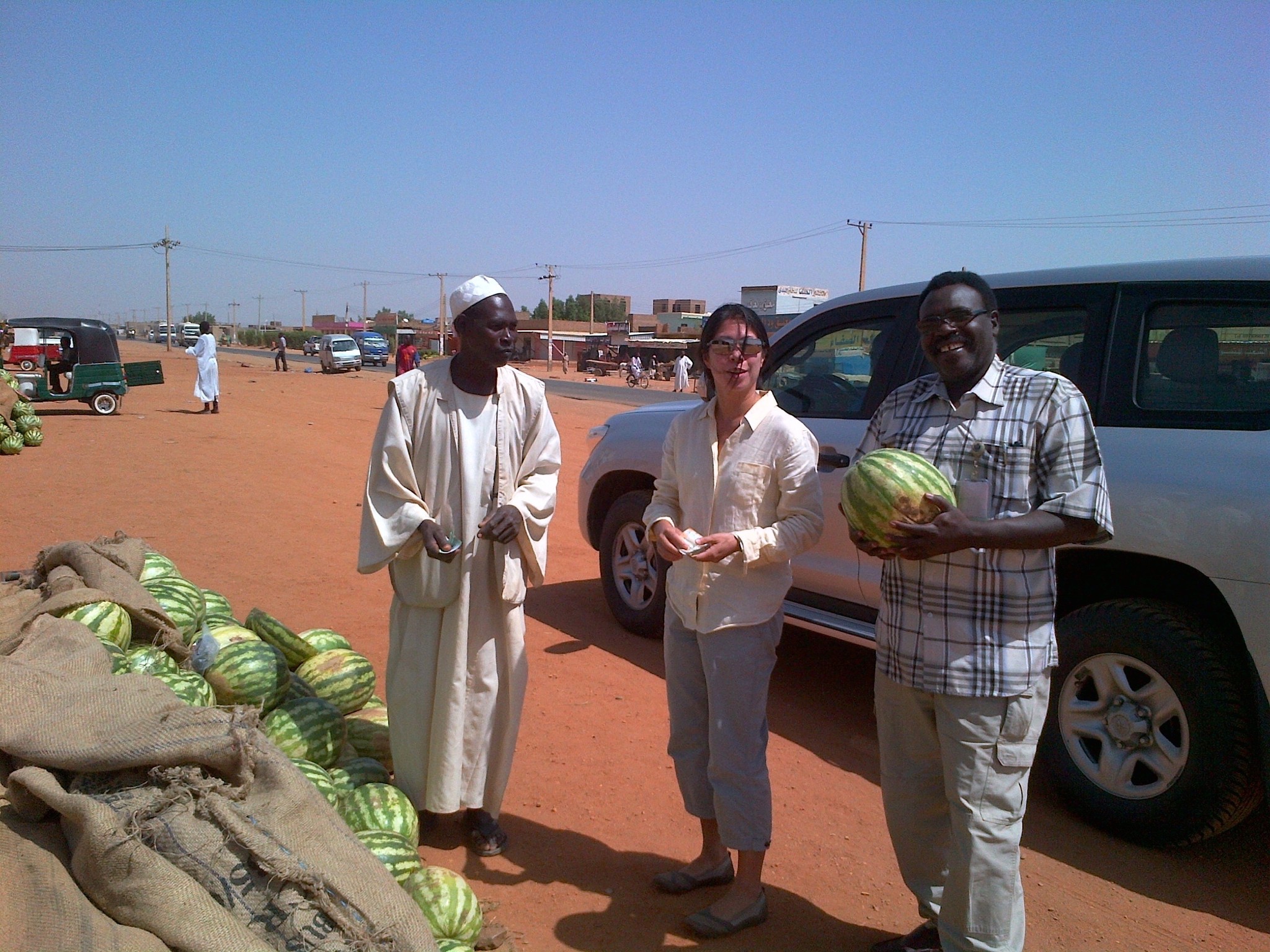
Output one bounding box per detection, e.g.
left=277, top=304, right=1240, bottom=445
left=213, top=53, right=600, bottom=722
left=841, top=448, right=956, bottom=557
left=55, top=547, right=482, bottom=952
left=0, top=369, right=44, bottom=455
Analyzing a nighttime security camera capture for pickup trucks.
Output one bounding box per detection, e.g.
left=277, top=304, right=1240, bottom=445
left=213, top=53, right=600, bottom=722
left=302, top=335, right=321, bottom=357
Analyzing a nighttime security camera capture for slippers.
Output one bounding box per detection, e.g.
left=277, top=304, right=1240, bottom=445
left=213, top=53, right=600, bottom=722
left=420, top=810, right=438, bottom=833
left=468, top=805, right=505, bottom=856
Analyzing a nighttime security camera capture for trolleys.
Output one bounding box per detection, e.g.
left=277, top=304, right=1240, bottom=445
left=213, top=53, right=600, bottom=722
left=653, top=361, right=676, bottom=381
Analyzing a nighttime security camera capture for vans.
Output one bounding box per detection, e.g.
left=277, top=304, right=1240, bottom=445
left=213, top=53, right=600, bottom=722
left=176, top=321, right=202, bottom=348
left=351, top=330, right=390, bottom=367
left=153, top=321, right=177, bottom=343
left=318, top=333, right=362, bottom=374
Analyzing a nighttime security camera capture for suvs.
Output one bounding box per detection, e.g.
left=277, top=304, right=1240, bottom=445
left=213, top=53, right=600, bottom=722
left=574, top=252, right=1270, bottom=853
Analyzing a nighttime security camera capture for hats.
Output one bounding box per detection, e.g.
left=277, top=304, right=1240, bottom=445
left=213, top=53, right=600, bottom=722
left=447, top=274, right=508, bottom=321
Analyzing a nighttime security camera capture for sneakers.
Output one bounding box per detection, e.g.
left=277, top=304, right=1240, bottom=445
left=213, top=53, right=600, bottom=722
left=870, top=921, right=943, bottom=952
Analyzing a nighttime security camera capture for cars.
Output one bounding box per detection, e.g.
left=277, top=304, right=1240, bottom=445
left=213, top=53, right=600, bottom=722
left=148, top=329, right=154, bottom=341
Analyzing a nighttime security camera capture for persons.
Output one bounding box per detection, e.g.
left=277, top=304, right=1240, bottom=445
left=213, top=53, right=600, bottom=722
left=220, top=331, right=226, bottom=339
left=271, top=333, right=288, bottom=372
left=671, top=351, right=694, bottom=392
left=396, top=335, right=420, bottom=378
left=562, top=352, right=570, bottom=373
left=129, top=330, right=134, bottom=340
left=629, top=352, right=644, bottom=383
left=648, top=355, right=657, bottom=380
left=356, top=274, right=563, bottom=857
left=49, top=337, right=77, bottom=394
left=272, top=339, right=276, bottom=348
left=185, top=321, right=219, bottom=413
left=836, top=271, right=1112, bottom=952
left=643, top=304, right=826, bottom=937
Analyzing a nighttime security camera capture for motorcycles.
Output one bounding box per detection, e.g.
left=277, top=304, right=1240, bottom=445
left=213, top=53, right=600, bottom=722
left=0, top=315, right=164, bottom=416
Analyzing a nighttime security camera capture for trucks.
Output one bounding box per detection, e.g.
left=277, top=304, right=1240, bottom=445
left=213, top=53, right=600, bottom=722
left=125, top=328, right=136, bottom=340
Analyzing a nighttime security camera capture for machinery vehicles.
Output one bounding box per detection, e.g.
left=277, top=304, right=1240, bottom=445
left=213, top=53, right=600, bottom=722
left=218, top=335, right=233, bottom=347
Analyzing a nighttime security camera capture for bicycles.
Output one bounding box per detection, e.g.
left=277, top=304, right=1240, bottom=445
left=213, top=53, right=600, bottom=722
left=625, top=368, right=649, bottom=389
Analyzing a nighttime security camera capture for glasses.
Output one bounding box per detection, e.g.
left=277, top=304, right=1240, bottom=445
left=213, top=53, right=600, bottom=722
left=915, top=307, right=994, bottom=335
left=706, top=339, right=764, bottom=357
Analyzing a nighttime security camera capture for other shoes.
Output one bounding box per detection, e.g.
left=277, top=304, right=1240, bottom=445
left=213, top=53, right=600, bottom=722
left=211, top=409, right=219, bottom=414
left=49, top=386, right=63, bottom=395
left=653, top=847, right=736, bottom=893
left=684, top=884, right=768, bottom=938
left=197, top=409, right=211, bottom=414
